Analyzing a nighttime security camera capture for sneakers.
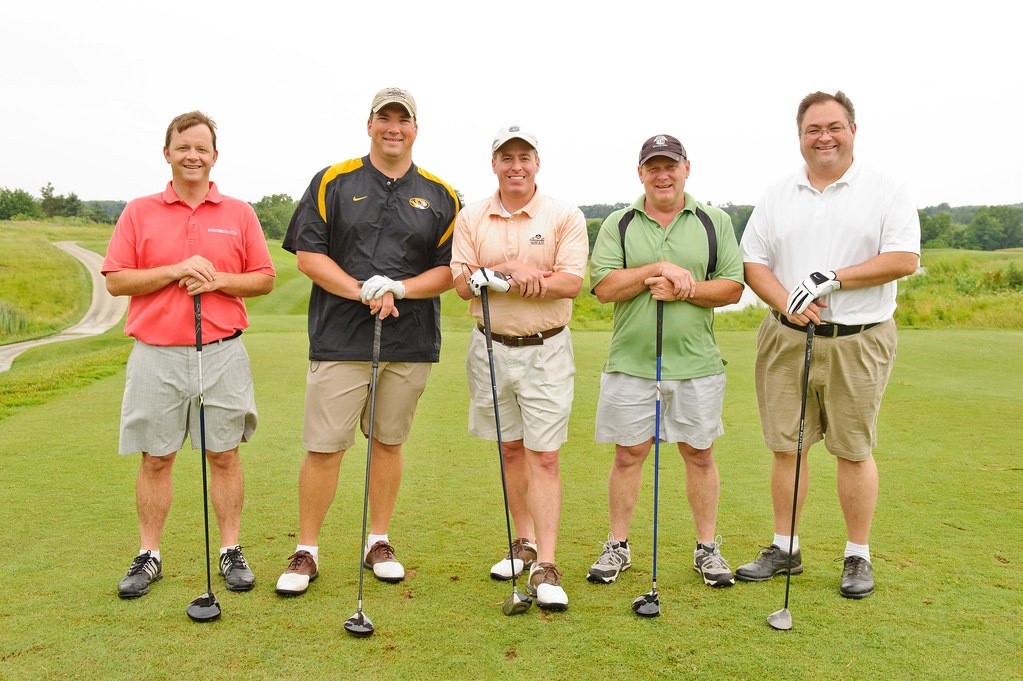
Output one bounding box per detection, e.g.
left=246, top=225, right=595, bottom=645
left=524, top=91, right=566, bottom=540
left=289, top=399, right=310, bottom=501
left=490, top=538, right=537, bottom=580
left=363, top=541, right=406, bottom=584
left=586, top=540, right=633, bottom=585
left=840, top=555, right=875, bottom=598
left=218, top=548, right=254, bottom=591
left=275, top=551, right=321, bottom=597
left=526, top=563, right=570, bottom=610
left=693, top=542, right=735, bottom=587
left=736, top=544, right=804, bottom=580
left=118, top=552, right=164, bottom=598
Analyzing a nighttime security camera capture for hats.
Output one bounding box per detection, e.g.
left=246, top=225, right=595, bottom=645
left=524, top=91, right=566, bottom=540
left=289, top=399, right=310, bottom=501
left=371, top=87, right=417, bottom=122
left=638, top=134, right=686, bottom=166
left=492, top=126, right=539, bottom=154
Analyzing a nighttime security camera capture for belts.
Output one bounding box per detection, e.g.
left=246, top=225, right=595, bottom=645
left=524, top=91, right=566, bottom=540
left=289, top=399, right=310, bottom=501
left=477, top=322, right=565, bottom=348
left=193, top=329, right=243, bottom=347
left=772, top=309, right=880, bottom=337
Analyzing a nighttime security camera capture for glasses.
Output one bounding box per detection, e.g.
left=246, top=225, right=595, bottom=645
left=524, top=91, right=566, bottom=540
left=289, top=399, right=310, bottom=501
left=801, top=122, right=850, bottom=139
left=462, top=261, right=472, bottom=286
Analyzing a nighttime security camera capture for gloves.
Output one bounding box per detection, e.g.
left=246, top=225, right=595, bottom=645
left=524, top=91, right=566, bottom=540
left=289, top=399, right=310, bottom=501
left=467, top=268, right=511, bottom=298
left=357, top=274, right=407, bottom=305
left=786, top=270, right=842, bottom=315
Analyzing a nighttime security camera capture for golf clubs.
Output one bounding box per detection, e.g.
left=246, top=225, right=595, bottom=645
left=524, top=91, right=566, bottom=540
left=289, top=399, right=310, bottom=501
left=480, top=284, right=532, bottom=615
left=767, top=296, right=819, bottom=631
left=344, top=310, right=383, bottom=636
left=632, top=299, right=665, bottom=616
left=185, top=293, right=222, bottom=623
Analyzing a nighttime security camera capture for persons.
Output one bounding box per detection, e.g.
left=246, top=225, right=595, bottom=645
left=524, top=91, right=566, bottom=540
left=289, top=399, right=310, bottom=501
left=276, top=87, right=461, bottom=597
left=450, top=126, right=588, bottom=612
left=587, top=136, right=747, bottom=586
left=101, top=112, right=276, bottom=599
left=736, top=91, right=921, bottom=599
